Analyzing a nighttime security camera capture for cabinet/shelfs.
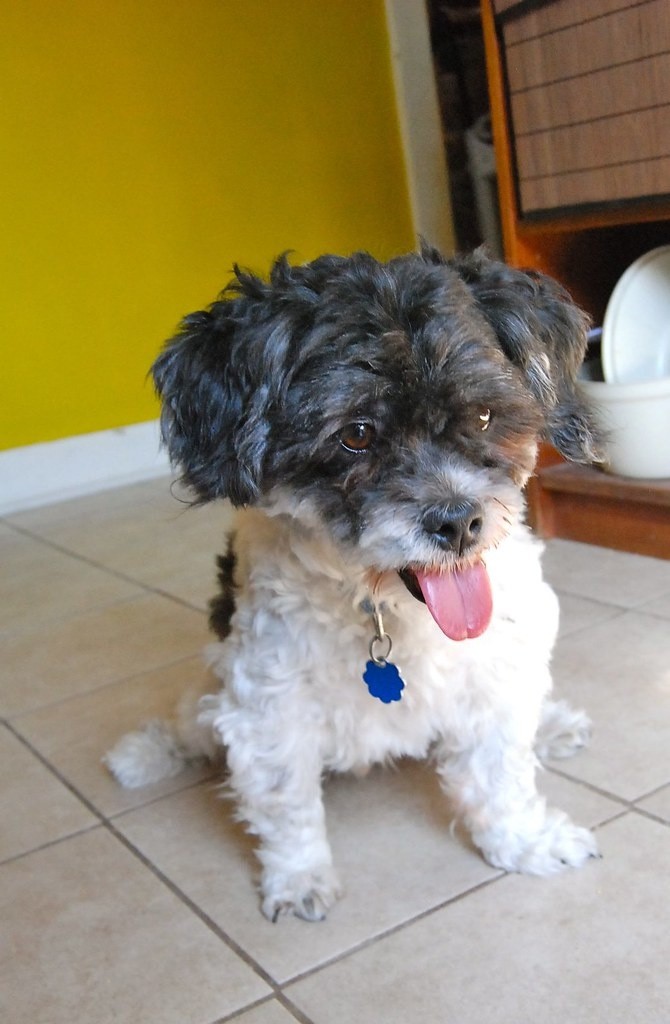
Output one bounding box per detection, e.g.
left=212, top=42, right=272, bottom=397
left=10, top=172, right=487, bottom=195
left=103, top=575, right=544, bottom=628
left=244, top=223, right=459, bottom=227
left=479, top=0, right=670, bottom=564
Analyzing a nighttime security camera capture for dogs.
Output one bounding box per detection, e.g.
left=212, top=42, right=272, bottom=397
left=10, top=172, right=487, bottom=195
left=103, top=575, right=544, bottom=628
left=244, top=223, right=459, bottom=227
left=98, top=235, right=611, bottom=919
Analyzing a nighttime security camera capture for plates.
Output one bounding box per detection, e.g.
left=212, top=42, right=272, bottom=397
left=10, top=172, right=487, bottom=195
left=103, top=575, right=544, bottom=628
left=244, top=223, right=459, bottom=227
left=601, top=244, right=670, bottom=382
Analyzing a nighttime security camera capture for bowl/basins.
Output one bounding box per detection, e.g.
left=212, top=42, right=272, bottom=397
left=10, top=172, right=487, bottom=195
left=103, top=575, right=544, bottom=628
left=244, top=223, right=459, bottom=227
left=576, top=360, right=670, bottom=479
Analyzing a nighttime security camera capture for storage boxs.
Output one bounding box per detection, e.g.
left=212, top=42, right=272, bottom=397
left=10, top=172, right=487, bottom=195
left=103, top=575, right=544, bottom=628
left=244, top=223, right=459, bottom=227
left=494, top=0, right=670, bottom=217
